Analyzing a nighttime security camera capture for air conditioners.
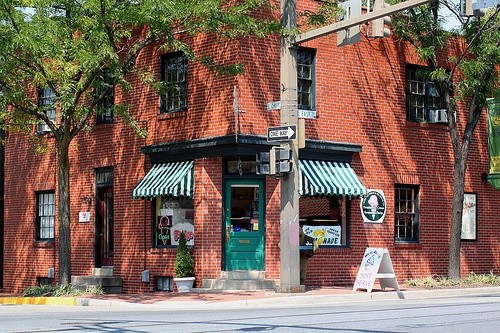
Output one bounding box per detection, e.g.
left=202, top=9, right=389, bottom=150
left=435, top=110, right=456, bottom=123
left=37, top=120, right=54, bottom=132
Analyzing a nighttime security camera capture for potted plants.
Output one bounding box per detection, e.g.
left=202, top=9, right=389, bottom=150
left=173, top=231, right=195, bottom=292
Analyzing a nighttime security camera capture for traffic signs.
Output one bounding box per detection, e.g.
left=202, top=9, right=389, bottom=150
left=266, top=125, right=297, bottom=143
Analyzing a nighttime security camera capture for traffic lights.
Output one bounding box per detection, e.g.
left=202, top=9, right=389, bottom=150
left=372, top=0, right=392, bottom=38
left=276, top=148, right=293, bottom=173
left=256, top=151, right=269, bottom=175
left=464, top=0, right=487, bottom=18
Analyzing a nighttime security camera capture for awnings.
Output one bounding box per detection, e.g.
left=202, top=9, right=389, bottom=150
left=132, top=160, right=194, bottom=200
left=297, top=159, right=367, bottom=196
